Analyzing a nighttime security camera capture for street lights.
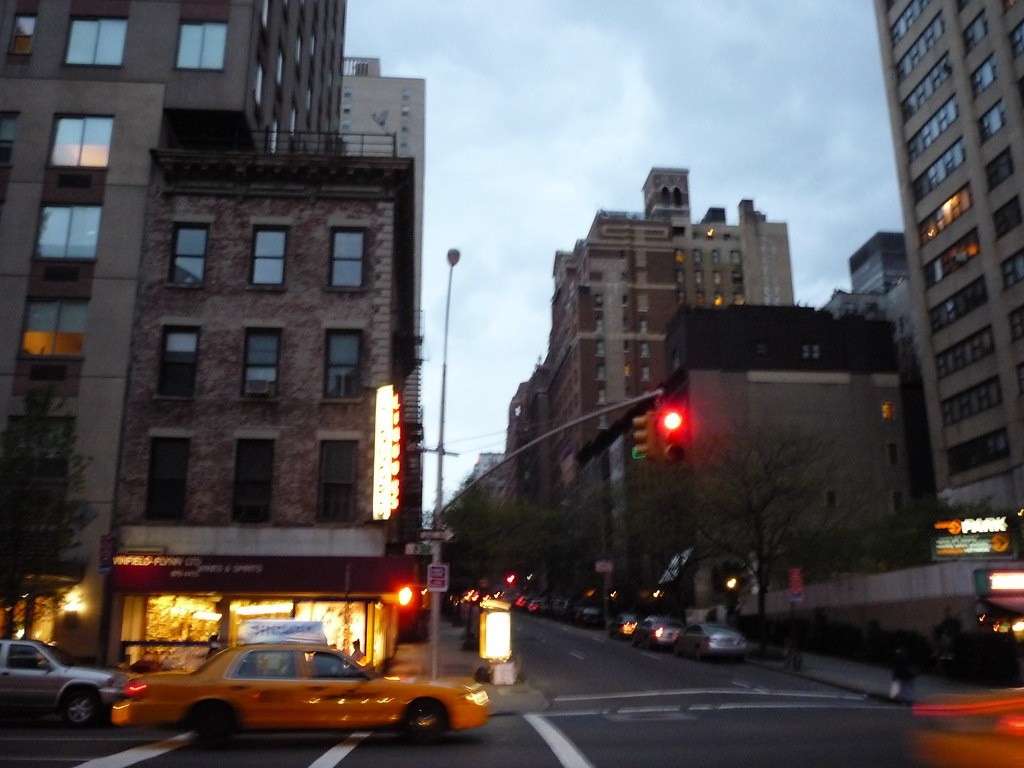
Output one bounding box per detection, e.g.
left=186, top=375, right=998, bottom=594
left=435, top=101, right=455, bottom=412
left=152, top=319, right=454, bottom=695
left=430, top=248, right=461, bottom=681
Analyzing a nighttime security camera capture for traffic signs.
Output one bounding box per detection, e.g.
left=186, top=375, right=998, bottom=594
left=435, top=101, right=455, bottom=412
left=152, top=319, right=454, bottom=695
left=419, top=528, right=456, bottom=542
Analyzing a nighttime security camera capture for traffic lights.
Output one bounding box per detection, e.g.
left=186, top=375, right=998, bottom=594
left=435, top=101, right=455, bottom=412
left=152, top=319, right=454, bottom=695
left=656, top=405, right=687, bottom=465
left=631, top=411, right=656, bottom=463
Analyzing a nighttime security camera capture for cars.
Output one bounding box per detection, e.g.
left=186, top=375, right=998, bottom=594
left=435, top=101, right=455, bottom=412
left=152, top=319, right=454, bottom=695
left=111, top=619, right=491, bottom=747
left=486, top=589, right=605, bottom=629
left=906, top=688, right=1024, bottom=768
left=631, top=615, right=686, bottom=653
left=0, top=638, right=130, bottom=730
left=608, top=613, right=640, bottom=640
left=671, top=623, right=749, bottom=662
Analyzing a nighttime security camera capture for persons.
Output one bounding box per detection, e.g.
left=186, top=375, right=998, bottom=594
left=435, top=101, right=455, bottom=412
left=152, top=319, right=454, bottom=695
left=206, top=633, right=223, bottom=658
left=350, top=639, right=367, bottom=664
